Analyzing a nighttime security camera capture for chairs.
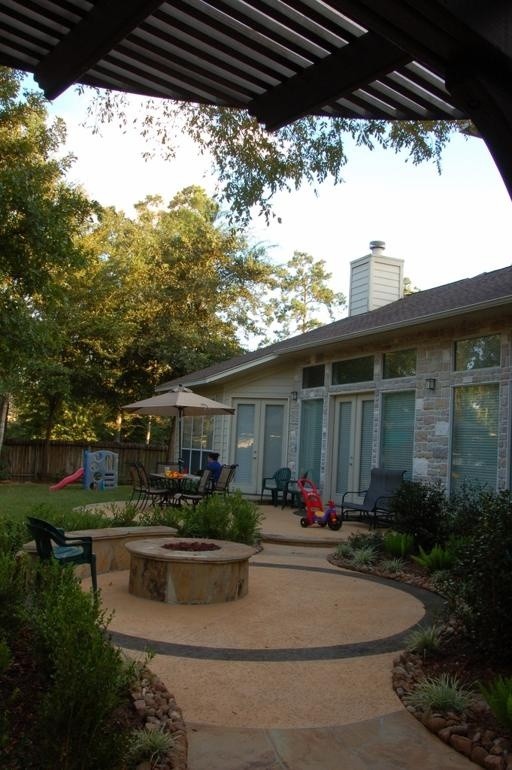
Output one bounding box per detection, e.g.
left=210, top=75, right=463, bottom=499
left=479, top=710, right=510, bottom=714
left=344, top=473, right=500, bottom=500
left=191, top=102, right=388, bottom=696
left=26, top=516, right=97, bottom=608
left=281, top=471, right=308, bottom=511
left=341, top=468, right=407, bottom=531
left=129, top=462, right=239, bottom=513
left=260, top=467, right=291, bottom=507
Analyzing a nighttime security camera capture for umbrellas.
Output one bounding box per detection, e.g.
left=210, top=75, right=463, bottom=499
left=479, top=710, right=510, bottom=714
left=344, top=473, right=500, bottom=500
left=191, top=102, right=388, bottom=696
left=120, top=383, right=236, bottom=473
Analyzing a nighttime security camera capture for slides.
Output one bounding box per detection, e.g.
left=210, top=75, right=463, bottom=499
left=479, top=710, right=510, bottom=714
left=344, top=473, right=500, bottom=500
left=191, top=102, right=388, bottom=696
left=49, top=468, right=84, bottom=491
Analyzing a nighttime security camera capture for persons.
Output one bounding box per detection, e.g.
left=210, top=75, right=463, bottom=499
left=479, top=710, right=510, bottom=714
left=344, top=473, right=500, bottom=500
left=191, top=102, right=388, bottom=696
left=197, top=453, right=222, bottom=484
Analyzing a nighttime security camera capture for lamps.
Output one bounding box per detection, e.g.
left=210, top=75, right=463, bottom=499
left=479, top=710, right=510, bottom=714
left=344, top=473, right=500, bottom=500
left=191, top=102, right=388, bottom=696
left=290, top=391, right=297, bottom=400
left=425, top=378, right=436, bottom=390
